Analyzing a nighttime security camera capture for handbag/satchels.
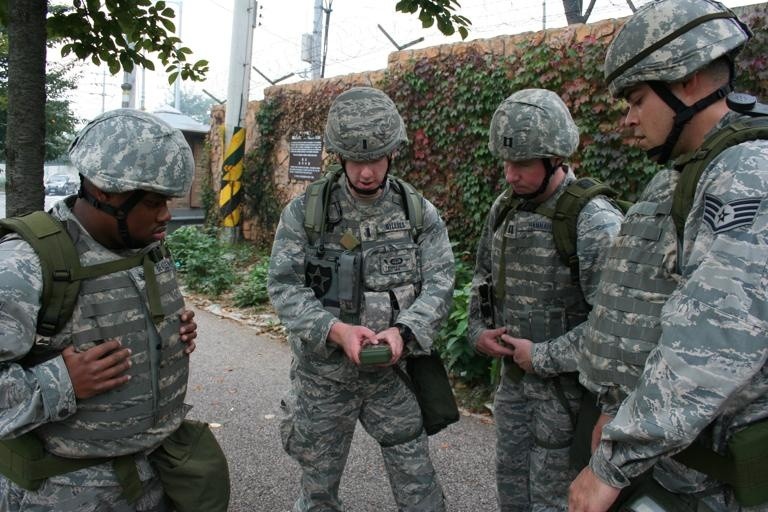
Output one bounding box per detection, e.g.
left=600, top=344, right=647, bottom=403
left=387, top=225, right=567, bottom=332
left=714, top=418, right=768, bottom=512
left=407, top=348, right=461, bottom=438
left=151, top=419, right=231, bottom=511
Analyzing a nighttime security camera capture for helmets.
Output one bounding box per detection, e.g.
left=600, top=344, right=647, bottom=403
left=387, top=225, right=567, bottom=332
left=486, top=85, right=581, bottom=161
left=322, top=86, right=410, bottom=161
left=604, top=0, right=755, bottom=97
left=67, top=107, right=196, bottom=200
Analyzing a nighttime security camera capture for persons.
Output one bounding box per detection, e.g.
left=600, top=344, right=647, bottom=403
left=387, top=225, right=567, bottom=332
left=268, top=86, right=456, bottom=512
left=466, top=87, right=626, bottom=512
left=0, top=108, right=198, bottom=512
left=569, top=0, right=768, bottom=512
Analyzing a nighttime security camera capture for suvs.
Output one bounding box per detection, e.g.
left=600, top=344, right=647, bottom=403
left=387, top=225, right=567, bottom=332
left=43, top=173, right=77, bottom=195
